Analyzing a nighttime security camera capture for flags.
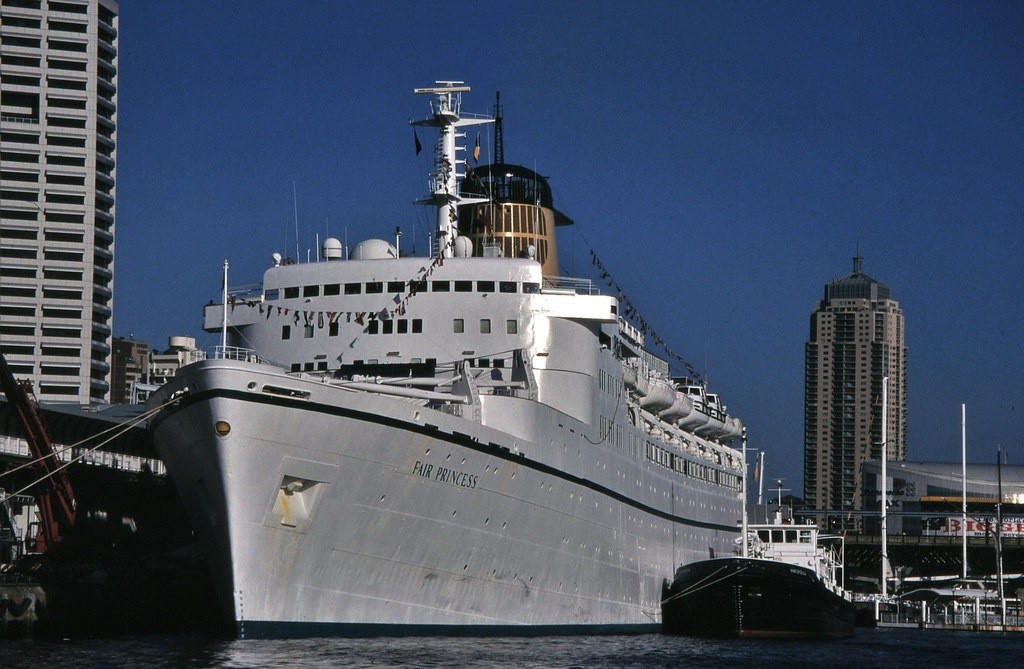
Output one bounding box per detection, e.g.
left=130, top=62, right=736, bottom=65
left=753, top=462, right=759, bottom=481
left=414, top=131, right=423, bottom=157
left=473, top=133, right=480, bottom=163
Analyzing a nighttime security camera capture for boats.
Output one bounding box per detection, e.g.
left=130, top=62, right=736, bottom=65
left=145, top=82, right=878, bottom=644
